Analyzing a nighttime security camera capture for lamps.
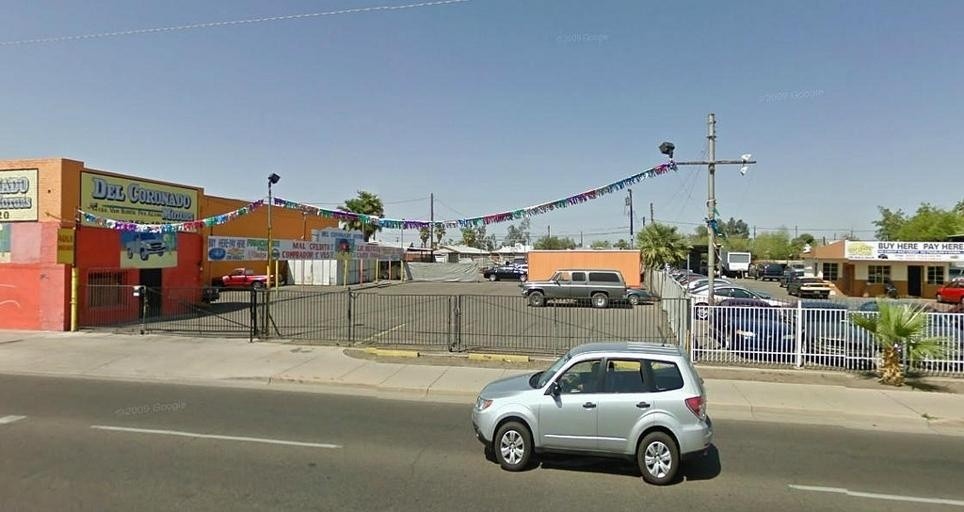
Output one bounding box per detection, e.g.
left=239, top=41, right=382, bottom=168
left=268, top=174, right=280, bottom=184
left=660, top=142, right=675, bottom=158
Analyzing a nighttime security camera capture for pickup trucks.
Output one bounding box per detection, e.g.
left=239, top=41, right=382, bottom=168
left=127, top=230, right=166, bottom=261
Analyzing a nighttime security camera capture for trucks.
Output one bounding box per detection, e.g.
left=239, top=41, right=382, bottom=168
left=211, top=267, right=281, bottom=289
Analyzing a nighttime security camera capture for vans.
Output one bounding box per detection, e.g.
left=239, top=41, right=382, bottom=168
left=467, top=337, right=715, bottom=488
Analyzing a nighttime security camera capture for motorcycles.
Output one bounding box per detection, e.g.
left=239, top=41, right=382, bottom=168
left=885, top=282, right=897, bottom=299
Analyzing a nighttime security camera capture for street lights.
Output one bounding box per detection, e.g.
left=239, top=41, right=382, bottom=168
left=265, top=170, right=282, bottom=287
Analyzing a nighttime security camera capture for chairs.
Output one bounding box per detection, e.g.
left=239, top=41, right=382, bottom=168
left=606, top=362, right=615, bottom=392
left=639, top=360, right=656, bottom=392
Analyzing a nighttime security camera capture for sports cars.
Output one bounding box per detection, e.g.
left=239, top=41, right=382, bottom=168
left=573, top=287, right=648, bottom=306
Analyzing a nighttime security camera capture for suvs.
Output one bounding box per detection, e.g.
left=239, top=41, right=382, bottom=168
left=520, top=266, right=628, bottom=309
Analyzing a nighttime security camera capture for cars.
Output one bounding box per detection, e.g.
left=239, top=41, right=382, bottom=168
left=857, top=299, right=964, bottom=369
left=935, top=265, right=964, bottom=324
left=482, top=257, right=528, bottom=284
left=665, top=256, right=876, bottom=366
